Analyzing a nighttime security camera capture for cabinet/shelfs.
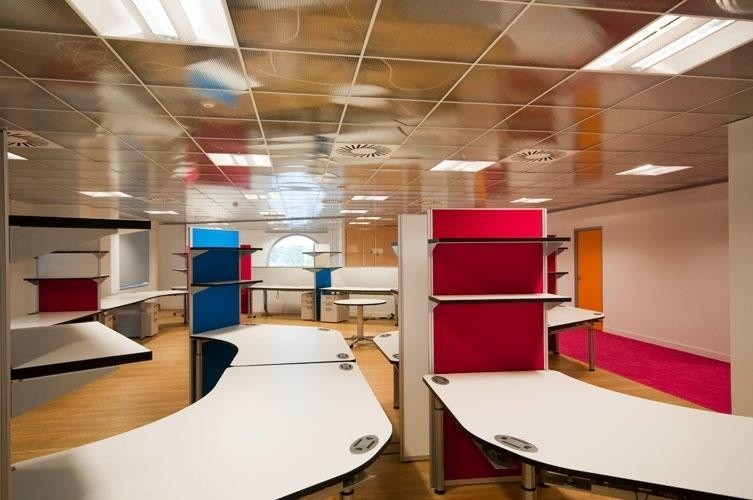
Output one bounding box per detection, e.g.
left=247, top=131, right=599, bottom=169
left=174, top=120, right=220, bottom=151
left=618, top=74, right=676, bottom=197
left=546, top=233, right=569, bottom=293
left=171, top=253, right=188, bottom=290
left=187, top=226, right=264, bottom=403
left=23, top=237, right=110, bottom=311
left=301, top=244, right=343, bottom=321
left=430, top=208, right=572, bottom=489
left=390, top=212, right=431, bottom=463
left=0, top=126, right=153, bottom=500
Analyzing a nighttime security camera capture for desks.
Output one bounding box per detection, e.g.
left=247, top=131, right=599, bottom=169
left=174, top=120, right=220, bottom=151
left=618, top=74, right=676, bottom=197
left=9, top=310, right=102, bottom=329
left=547, top=305, right=605, bottom=371
left=318, top=287, right=395, bottom=323
left=424, top=369, right=753, bottom=500
left=334, top=298, right=387, bottom=349
left=11, top=322, right=393, bottom=500
left=247, top=284, right=314, bottom=320
left=99, top=289, right=188, bottom=340
left=372, top=329, right=400, bottom=410
